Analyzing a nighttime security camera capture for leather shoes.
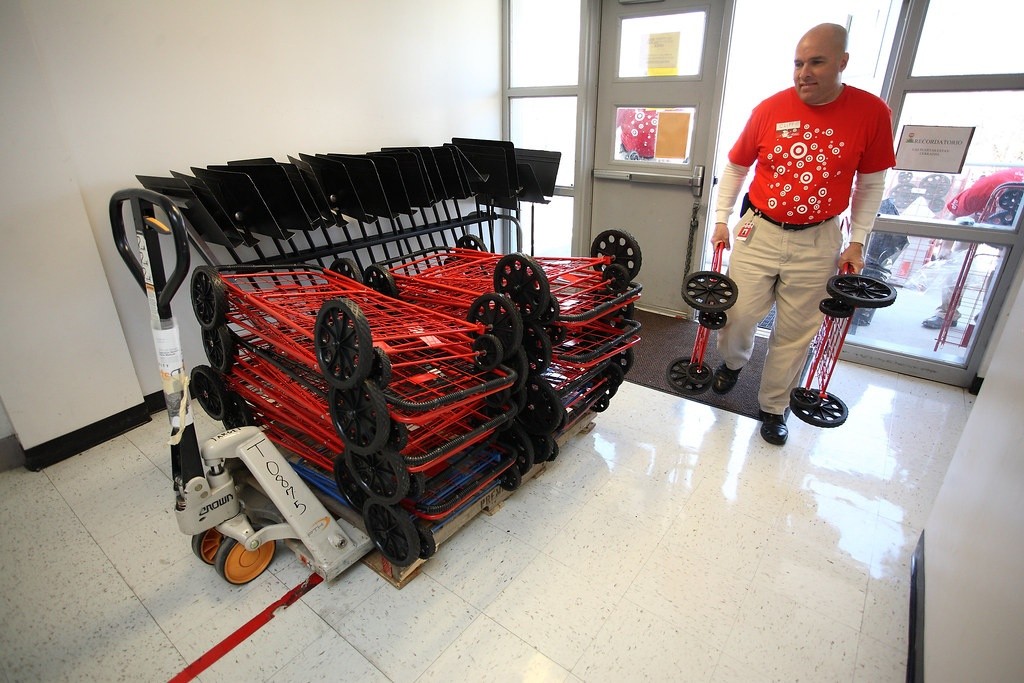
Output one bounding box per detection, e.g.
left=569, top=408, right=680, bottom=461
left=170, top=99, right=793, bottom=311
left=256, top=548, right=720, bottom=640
left=712, top=360, right=744, bottom=395
left=759, top=408, right=789, bottom=445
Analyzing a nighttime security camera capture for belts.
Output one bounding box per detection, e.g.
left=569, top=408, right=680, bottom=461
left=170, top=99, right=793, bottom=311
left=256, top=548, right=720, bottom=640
left=747, top=201, right=834, bottom=231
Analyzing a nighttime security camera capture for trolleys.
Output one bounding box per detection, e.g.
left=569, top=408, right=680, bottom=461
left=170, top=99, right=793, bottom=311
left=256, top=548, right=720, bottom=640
left=109, top=189, right=376, bottom=587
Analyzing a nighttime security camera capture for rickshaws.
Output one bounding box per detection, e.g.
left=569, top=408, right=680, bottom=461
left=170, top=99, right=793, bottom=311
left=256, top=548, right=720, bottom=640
left=789, top=217, right=899, bottom=429
left=666, top=232, right=738, bottom=399
left=190, top=228, right=644, bottom=563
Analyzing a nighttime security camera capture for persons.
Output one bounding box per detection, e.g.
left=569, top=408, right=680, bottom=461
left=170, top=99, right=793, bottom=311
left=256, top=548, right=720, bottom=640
left=710, top=23, right=897, bottom=446
left=616, top=68, right=683, bottom=164
left=923, top=167, right=1023, bottom=328
